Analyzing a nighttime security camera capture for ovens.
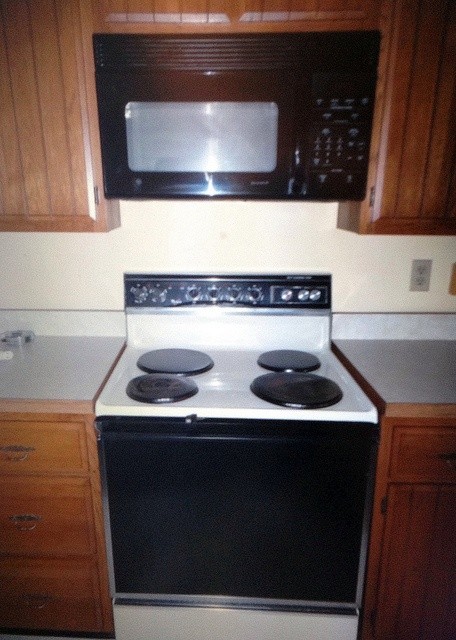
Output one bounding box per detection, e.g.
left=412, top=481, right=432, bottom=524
left=93, top=416, right=377, bottom=615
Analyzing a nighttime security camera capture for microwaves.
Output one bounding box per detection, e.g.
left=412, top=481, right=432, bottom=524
left=92, top=29, right=382, bottom=201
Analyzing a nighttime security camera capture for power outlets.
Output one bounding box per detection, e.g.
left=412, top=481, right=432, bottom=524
left=409, top=258, right=432, bottom=291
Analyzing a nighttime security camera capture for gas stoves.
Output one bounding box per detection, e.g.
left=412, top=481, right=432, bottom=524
left=94, top=345, right=379, bottom=425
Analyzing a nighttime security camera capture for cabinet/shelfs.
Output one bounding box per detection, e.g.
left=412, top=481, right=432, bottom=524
left=86, top=0, right=385, bottom=35
left=359, top=418, right=456, bottom=640
left=0, top=0, right=120, bottom=233
left=337, top=0, right=455, bottom=237
left=0, top=411, right=113, bottom=638
